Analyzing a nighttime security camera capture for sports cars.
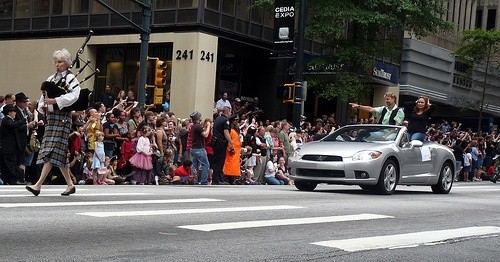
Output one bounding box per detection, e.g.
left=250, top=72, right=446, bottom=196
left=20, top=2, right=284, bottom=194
left=288, top=124, right=456, bottom=195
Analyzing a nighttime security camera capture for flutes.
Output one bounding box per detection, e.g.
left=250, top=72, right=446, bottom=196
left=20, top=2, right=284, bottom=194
left=57, top=29, right=100, bottom=94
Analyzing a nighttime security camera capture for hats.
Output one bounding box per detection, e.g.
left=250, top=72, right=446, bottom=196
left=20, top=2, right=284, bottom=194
left=12, top=92, right=30, bottom=102
left=1, top=104, right=17, bottom=113
left=190, top=111, right=202, bottom=123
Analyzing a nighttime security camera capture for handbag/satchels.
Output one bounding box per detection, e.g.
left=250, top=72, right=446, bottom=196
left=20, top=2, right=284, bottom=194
left=30, top=132, right=40, bottom=153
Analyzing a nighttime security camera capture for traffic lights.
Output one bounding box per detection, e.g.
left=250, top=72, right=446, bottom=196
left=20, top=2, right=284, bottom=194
left=154, top=60, right=167, bottom=86
left=282, top=83, right=295, bottom=104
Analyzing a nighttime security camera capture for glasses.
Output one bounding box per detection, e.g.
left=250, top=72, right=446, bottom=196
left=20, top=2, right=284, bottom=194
left=20, top=100, right=29, bottom=103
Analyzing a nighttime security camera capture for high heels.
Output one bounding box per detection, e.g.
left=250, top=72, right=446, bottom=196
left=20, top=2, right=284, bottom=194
left=26, top=185, right=41, bottom=197
left=61, top=186, right=76, bottom=196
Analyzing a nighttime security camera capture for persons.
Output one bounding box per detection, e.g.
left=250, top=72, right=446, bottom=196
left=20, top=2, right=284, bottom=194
left=0, top=84, right=500, bottom=185
left=26, top=48, right=75, bottom=197
